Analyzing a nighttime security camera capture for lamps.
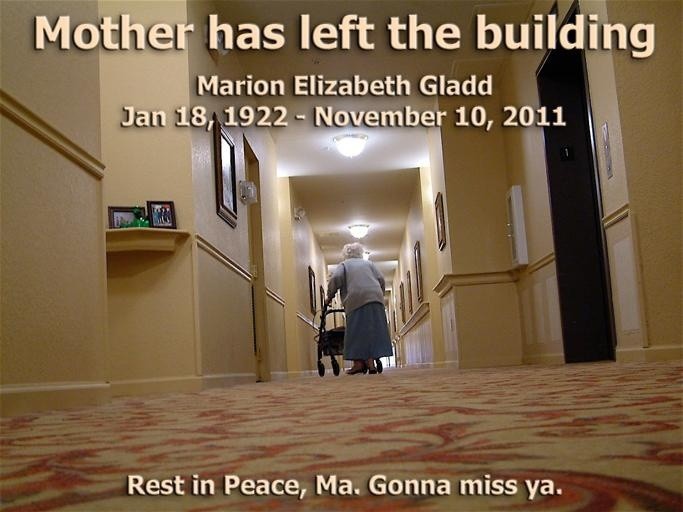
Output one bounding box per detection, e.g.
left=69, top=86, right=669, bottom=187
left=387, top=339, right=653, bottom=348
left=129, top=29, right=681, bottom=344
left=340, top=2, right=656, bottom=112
left=239, top=180, right=258, bottom=205
left=348, top=224, right=369, bottom=240
left=334, top=132, right=367, bottom=159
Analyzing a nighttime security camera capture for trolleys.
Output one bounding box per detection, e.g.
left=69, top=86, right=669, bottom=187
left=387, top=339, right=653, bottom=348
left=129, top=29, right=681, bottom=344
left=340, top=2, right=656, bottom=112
left=313, top=285, right=383, bottom=377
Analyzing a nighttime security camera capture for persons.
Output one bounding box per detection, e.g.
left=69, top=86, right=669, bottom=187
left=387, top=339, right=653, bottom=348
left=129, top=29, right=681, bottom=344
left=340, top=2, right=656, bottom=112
left=327, top=240, right=394, bottom=376
left=153, top=205, right=172, bottom=225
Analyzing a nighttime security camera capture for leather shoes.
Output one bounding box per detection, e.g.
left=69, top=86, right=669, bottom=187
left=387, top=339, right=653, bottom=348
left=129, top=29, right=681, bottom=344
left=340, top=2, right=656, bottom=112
left=346, top=367, right=377, bottom=374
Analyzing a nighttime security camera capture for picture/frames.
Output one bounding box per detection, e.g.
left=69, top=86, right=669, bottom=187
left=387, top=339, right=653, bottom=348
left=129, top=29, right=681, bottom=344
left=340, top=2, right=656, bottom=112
left=146, top=200, right=176, bottom=229
left=107, top=206, right=145, bottom=229
left=399, top=193, right=446, bottom=324
left=212, top=111, right=238, bottom=230
left=309, top=266, right=316, bottom=316
left=321, top=285, right=325, bottom=309
